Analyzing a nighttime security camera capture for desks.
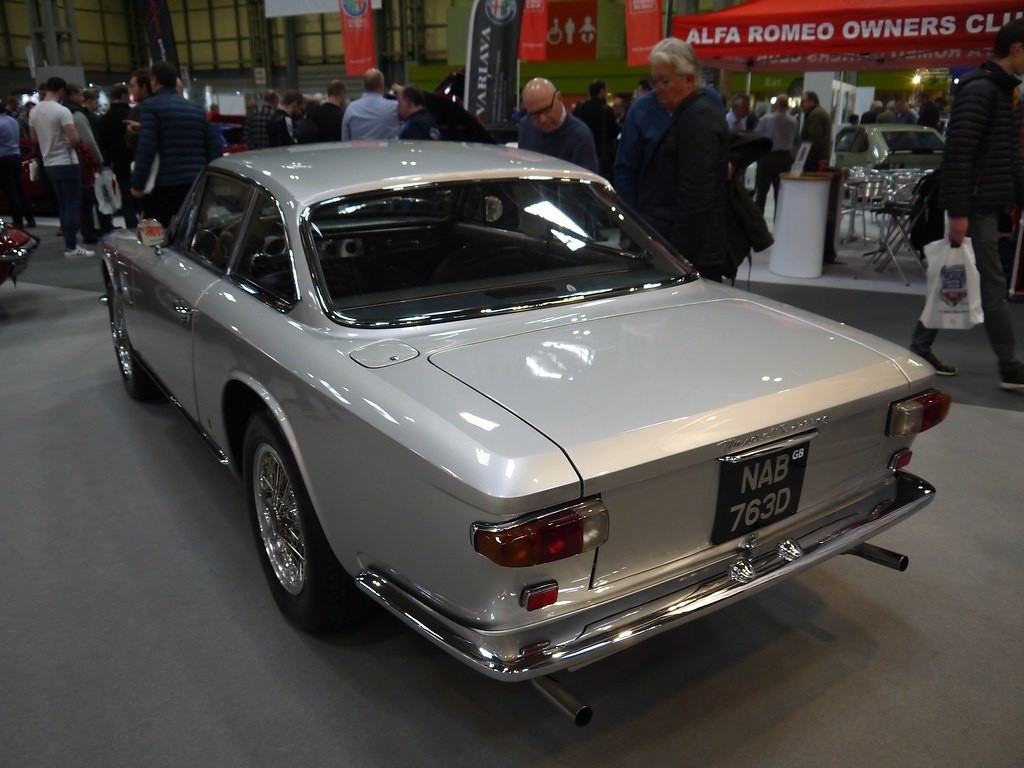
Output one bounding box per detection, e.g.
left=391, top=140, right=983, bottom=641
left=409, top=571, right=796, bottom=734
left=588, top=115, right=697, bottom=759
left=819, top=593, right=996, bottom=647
left=841, top=176, right=883, bottom=244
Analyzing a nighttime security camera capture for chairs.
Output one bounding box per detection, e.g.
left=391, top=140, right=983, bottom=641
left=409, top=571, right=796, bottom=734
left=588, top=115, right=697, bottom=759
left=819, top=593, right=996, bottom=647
left=840, top=165, right=939, bottom=286
left=237, top=236, right=296, bottom=298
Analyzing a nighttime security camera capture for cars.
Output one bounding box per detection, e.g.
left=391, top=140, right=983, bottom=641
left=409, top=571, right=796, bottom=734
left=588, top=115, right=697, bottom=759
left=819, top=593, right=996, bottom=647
left=100, top=141, right=954, bottom=727
left=834, top=123, right=946, bottom=173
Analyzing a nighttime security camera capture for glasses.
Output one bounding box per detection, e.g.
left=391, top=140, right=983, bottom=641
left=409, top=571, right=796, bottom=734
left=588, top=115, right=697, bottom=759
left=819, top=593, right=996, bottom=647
left=528, top=89, right=558, bottom=120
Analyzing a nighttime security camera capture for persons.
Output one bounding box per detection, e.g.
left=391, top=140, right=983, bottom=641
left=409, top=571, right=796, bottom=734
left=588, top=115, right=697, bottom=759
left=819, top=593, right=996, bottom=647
left=245, top=67, right=447, bottom=150
left=516, top=38, right=943, bottom=283
left=0, top=60, right=221, bottom=259
left=911, top=19, right=1024, bottom=391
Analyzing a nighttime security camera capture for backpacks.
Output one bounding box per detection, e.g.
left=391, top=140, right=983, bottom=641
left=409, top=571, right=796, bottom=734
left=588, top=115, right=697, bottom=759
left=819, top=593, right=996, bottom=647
left=908, top=166, right=946, bottom=254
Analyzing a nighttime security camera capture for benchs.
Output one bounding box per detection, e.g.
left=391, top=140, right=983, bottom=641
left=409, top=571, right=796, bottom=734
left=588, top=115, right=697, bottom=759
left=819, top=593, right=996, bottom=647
left=332, top=263, right=627, bottom=312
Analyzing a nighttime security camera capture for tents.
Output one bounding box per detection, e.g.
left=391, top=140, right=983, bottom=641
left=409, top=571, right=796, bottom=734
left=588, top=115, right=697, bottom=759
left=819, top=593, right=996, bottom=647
left=674, top=0, right=1024, bottom=98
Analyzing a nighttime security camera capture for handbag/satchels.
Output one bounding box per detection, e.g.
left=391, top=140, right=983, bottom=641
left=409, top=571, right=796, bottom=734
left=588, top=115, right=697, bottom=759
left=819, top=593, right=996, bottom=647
left=918, top=234, right=984, bottom=329
left=93, top=167, right=122, bottom=214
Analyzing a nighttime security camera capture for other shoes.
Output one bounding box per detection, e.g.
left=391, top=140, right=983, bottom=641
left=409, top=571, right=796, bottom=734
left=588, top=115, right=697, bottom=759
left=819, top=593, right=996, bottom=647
left=25, top=223, right=37, bottom=228
left=56, top=225, right=63, bottom=236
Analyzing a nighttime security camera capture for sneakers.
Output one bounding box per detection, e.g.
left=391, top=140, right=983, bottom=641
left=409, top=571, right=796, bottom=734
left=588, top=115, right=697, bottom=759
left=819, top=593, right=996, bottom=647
left=64, top=244, right=95, bottom=258
left=997, top=360, right=1024, bottom=388
left=910, top=349, right=957, bottom=375
